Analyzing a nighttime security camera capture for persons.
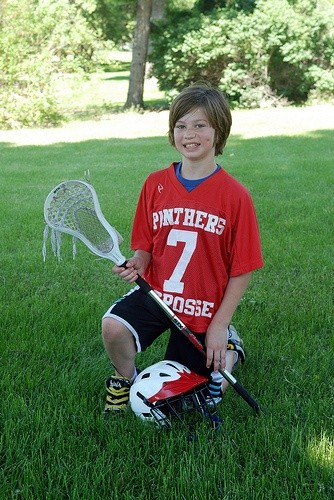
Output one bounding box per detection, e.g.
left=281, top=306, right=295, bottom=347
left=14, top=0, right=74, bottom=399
left=101, top=79, right=264, bottom=413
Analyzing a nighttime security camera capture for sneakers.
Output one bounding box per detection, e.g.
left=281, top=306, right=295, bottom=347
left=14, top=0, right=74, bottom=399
left=226, top=324, right=247, bottom=363
left=104, top=367, right=141, bottom=413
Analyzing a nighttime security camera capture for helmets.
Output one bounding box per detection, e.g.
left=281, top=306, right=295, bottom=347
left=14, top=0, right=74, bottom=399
left=129, top=360, right=216, bottom=429
